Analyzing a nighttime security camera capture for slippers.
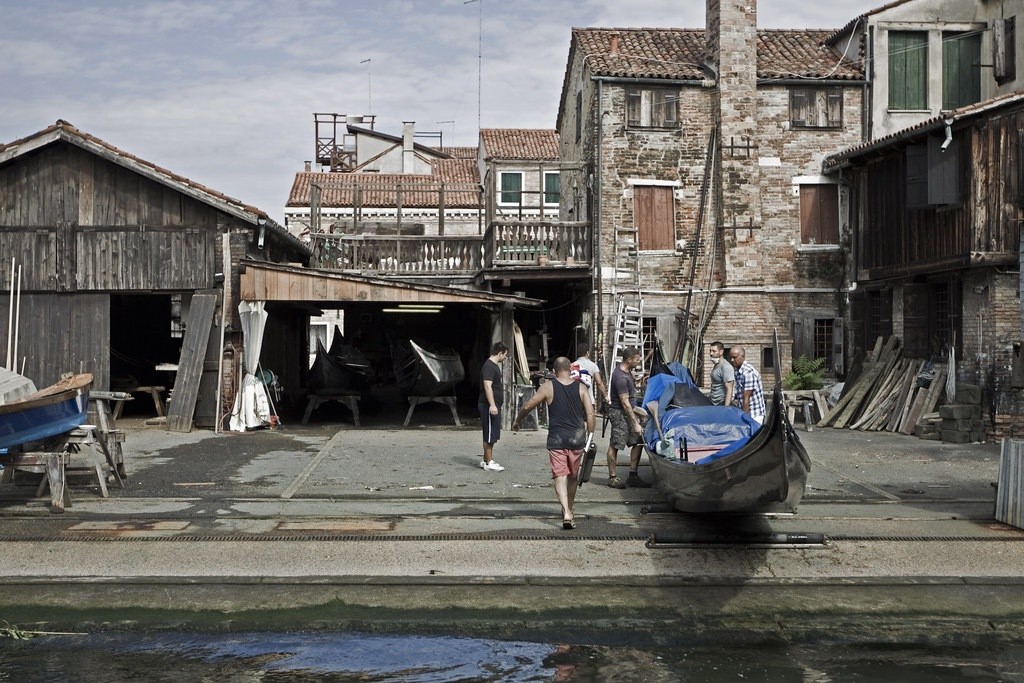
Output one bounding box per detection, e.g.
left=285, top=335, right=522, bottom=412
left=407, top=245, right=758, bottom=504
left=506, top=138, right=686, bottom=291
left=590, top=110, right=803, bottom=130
left=563, top=520, right=577, bottom=529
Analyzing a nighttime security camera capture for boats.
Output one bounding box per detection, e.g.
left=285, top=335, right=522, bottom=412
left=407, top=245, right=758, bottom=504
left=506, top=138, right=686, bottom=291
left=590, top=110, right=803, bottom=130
left=641, top=328, right=812, bottom=510
left=307, top=339, right=381, bottom=395
left=390, top=332, right=464, bottom=394
left=0, top=373, right=96, bottom=449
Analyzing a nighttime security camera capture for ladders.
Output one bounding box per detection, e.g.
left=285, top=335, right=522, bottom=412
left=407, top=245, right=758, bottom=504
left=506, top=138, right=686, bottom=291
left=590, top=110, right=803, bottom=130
left=607, top=224, right=645, bottom=406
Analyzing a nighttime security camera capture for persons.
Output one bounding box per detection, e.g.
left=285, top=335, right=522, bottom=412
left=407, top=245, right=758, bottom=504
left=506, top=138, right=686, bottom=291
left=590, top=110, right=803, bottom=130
left=478, top=341, right=508, bottom=472
left=512, top=355, right=595, bottom=529
left=729, top=345, right=766, bottom=425
left=606, top=346, right=651, bottom=489
left=569, top=343, right=607, bottom=466
left=709, top=340, right=734, bottom=406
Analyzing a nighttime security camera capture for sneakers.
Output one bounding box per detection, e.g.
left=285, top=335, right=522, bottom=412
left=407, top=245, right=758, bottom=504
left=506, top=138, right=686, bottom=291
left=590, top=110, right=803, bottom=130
left=479, top=460, right=504, bottom=472
left=627, top=475, right=651, bottom=488
left=607, top=475, right=627, bottom=489
